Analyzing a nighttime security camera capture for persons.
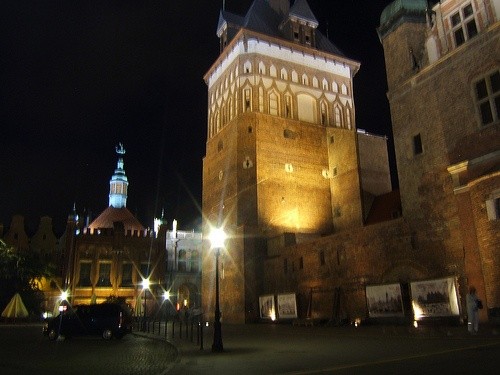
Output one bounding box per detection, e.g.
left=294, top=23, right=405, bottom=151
left=465, top=284, right=480, bottom=333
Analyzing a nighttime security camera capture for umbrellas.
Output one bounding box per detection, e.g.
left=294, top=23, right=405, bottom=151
left=0, top=292, right=29, bottom=319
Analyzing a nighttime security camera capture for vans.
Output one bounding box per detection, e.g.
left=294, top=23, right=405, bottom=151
left=41, top=302, right=134, bottom=341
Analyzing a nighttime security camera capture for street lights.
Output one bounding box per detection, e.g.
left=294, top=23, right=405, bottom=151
left=141, top=277, right=150, bottom=331
left=203, top=226, right=228, bottom=353
